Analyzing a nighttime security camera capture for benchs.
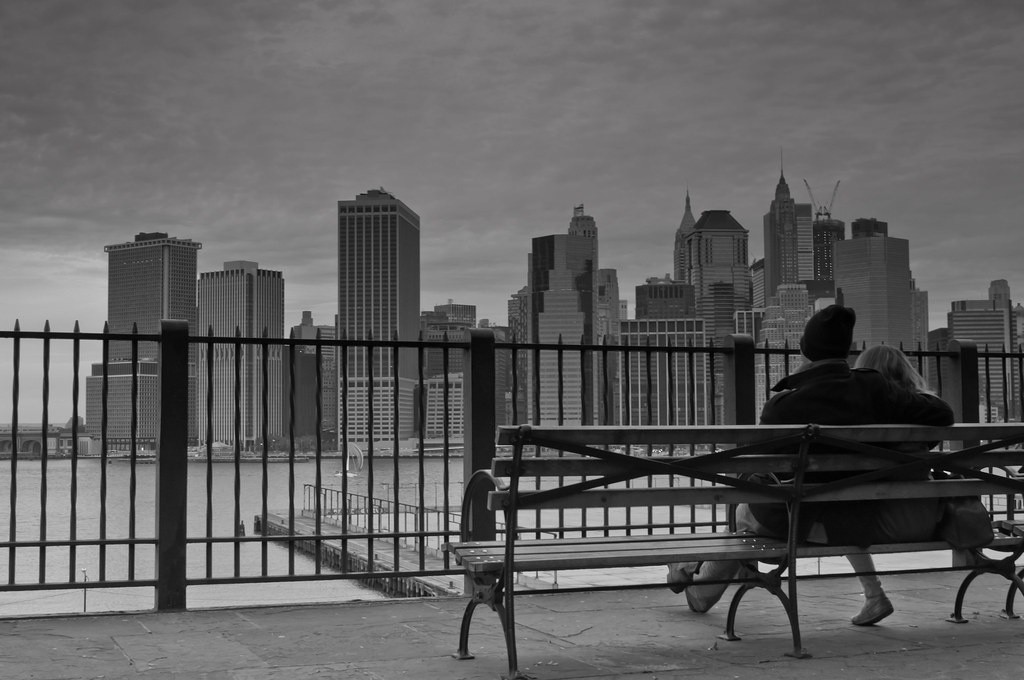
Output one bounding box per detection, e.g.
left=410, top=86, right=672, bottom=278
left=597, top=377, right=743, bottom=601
left=441, top=422, right=1024, bottom=680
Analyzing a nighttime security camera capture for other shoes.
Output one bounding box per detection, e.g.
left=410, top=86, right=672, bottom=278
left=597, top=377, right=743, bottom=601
left=851, top=597, right=894, bottom=625
left=683, top=587, right=698, bottom=612
left=666, top=562, right=690, bottom=593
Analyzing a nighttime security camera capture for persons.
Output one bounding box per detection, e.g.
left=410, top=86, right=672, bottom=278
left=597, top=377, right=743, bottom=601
left=668, top=304, right=956, bottom=613
left=845, top=345, right=938, bottom=624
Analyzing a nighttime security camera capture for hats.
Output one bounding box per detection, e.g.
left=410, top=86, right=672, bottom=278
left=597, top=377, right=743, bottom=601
left=800, top=305, right=856, bottom=360
